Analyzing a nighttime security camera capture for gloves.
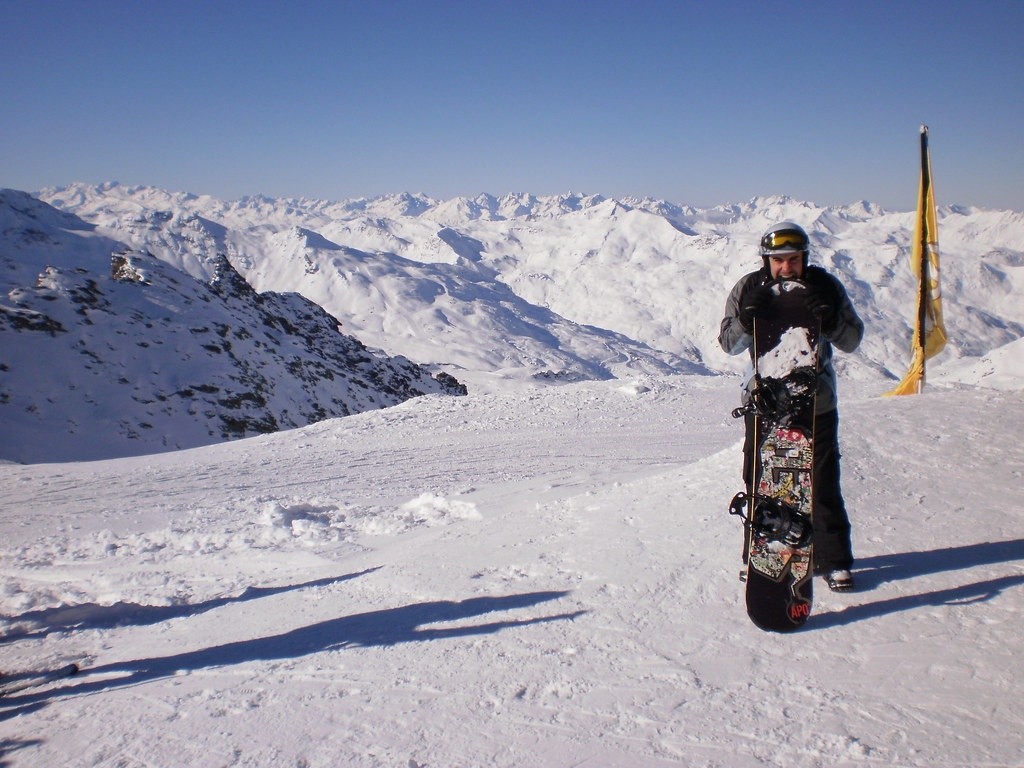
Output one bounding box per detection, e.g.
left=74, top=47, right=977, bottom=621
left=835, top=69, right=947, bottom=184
left=780, top=277, right=823, bottom=312
left=739, top=280, right=781, bottom=329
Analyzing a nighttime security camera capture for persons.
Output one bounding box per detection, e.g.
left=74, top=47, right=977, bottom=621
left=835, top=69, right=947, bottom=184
left=719, top=222, right=854, bottom=594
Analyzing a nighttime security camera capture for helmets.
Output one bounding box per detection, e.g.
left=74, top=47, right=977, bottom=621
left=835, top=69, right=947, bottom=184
left=758, top=221, right=810, bottom=267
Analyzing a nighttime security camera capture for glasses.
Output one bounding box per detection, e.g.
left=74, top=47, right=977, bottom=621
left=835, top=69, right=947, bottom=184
left=760, top=229, right=810, bottom=250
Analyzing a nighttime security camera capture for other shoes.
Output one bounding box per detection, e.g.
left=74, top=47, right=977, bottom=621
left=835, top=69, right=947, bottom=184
left=826, top=565, right=853, bottom=590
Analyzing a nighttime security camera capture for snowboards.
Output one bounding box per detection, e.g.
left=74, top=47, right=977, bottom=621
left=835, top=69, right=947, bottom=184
left=745, top=277, right=820, bottom=631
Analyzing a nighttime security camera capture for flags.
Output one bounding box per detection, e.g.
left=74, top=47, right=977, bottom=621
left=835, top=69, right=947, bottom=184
left=889, top=136, right=949, bottom=396
